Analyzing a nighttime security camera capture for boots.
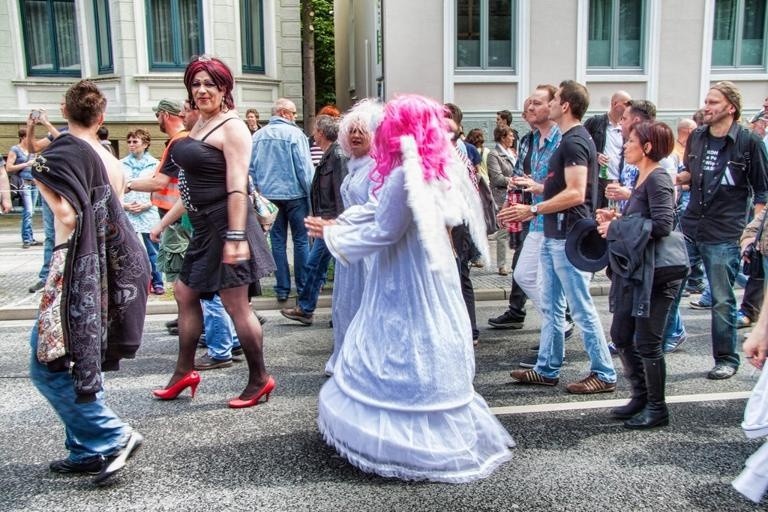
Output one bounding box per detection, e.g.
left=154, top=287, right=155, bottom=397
left=567, top=374, right=617, bottom=394
left=624, top=357, right=668, bottom=429
left=613, top=346, right=646, bottom=422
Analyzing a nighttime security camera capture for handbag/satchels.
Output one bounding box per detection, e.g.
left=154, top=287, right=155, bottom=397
left=10, top=176, right=23, bottom=196
left=248, top=184, right=280, bottom=240
left=652, top=226, right=692, bottom=282
left=743, top=247, right=764, bottom=277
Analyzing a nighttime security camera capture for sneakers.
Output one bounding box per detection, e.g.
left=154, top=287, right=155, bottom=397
left=530, top=326, right=573, bottom=352
left=21, top=240, right=42, bottom=250
left=29, top=281, right=46, bottom=293
left=511, top=369, right=560, bottom=387
left=520, top=354, right=566, bottom=368
left=155, top=287, right=164, bottom=295
left=706, top=363, right=737, bottom=383
left=166, top=312, right=267, bottom=347
left=488, top=310, right=526, bottom=329
left=665, top=331, right=687, bottom=354
left=49, top=458, right=104, bottom=475
left=606, top=341, right=622, bottom=354
left=690, top=300, right=712, bottom=309
left=473, top=334, right=479, bottom=346
left=97, top=431, right=144, bottom=488
left=281, top=306, right=314, bottom=326
left=500, top=266, right=508, bottom=277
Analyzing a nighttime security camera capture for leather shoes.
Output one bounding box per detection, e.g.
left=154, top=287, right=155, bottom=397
left=277, top=296, right=287, bottom=301
left=194, top=352, right=233, bottom=371
left=231, top=347, right=245, bottom=362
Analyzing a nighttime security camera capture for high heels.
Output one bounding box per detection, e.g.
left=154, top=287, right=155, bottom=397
left=228, top=375, right=278, bottom=408
left=152, top=370, right=202, bottom=400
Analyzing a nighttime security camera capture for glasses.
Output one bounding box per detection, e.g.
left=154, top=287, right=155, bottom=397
left=126, top=140, right=143, bottom=145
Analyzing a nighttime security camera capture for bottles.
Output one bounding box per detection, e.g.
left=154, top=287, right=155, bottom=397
left=599, top=152, right=609, bottom=180
left=507, top=189, right=524, bottom=234
left=607, top=178, right=619, bottom=213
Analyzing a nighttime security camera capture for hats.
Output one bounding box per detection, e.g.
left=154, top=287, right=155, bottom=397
left=151, top=99, right=182, bottom=116
left=565, top=218, right=609, bottom=273
left=708, top=80, right=744, bottom=118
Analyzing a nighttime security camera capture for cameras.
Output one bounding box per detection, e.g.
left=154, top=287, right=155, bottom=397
left=30, top=108, right=43, bottom=122
